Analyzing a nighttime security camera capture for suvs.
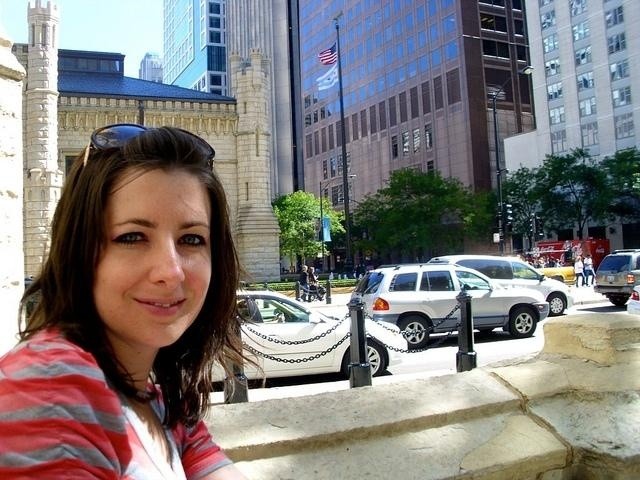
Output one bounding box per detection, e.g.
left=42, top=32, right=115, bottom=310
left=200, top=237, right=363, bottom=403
left=346, top=248, right=640, bottom=350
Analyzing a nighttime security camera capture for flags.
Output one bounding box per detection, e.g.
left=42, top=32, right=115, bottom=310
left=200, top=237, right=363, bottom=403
left=317, top=42, right=338, bottom=65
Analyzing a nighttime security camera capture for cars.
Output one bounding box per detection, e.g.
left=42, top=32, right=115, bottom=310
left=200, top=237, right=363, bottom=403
left=204, top=288, right=412, bottom=389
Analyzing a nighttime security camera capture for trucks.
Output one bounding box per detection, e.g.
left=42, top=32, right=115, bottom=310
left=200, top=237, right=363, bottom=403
left=532, top=237, right=610, bottom=272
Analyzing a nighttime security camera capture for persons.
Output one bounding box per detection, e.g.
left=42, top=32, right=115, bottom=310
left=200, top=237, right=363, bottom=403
left=353, top=264, right=366, bottom=280
left=1, top=125, right=250, bottom=480
left=525, top=256, right=560, bottom=269
left=309, top=267, right=329, bottom=302
left=574, top=256, right=586, bottom=287
left=583, top=254, right=596, bottom=286
left=300, top=265, right=310, bottom=301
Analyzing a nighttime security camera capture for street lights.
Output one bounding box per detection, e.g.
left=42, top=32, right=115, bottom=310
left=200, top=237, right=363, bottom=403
left=490, top=63, right=537, bottom=253
left=319, top=174, right=358, bottom=274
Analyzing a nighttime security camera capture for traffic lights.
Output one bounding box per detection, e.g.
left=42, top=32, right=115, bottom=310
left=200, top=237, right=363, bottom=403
left=529, top=213, right=534, bottom=234
left=503, top=202, right=515, bottom=230
left=532, top=212, right=545, bottom=238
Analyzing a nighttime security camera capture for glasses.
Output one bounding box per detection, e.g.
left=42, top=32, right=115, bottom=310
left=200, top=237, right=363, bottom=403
left=81, top=123, right=218, bottom=169
